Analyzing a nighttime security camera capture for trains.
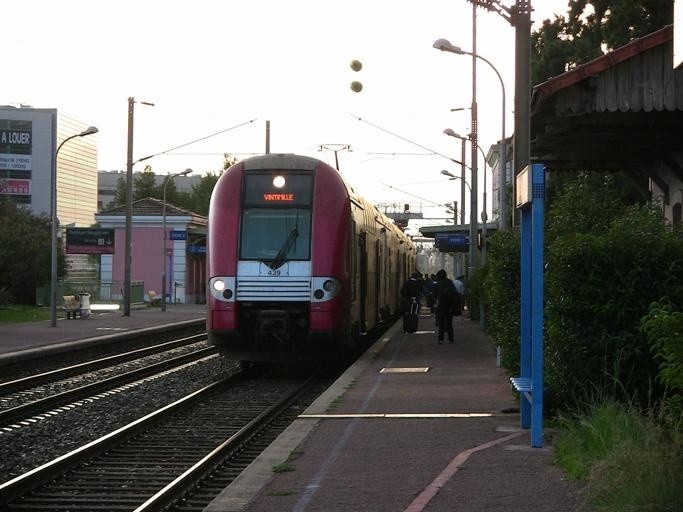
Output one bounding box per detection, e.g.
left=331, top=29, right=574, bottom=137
left=207, top=154, right=417, bottom=360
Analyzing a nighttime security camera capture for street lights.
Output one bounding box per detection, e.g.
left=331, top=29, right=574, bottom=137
left=434, top=39, right=505, bottom=368
left=161, top=168, right=193, bottom=312
left=51, top=126, right=99, bottom=328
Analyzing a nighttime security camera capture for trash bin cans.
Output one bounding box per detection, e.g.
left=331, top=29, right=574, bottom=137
left=78, top=292, right=92, bottom=319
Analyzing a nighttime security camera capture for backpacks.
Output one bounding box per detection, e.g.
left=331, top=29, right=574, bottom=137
left=446, top=286, right=461, bottom=316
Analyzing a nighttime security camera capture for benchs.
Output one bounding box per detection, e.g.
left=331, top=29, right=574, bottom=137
left=148, top=290, right=162, bottom=306
left=509, top=377, right=549, bottom=405
left=61, top=295, right=82, bottom=320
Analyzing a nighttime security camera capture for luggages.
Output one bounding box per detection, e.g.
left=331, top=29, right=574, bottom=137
left=403, top=313, right=418, bottom=334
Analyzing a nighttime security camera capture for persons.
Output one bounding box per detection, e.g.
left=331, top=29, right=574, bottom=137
left=400, top=268, right=465, bottom=345
